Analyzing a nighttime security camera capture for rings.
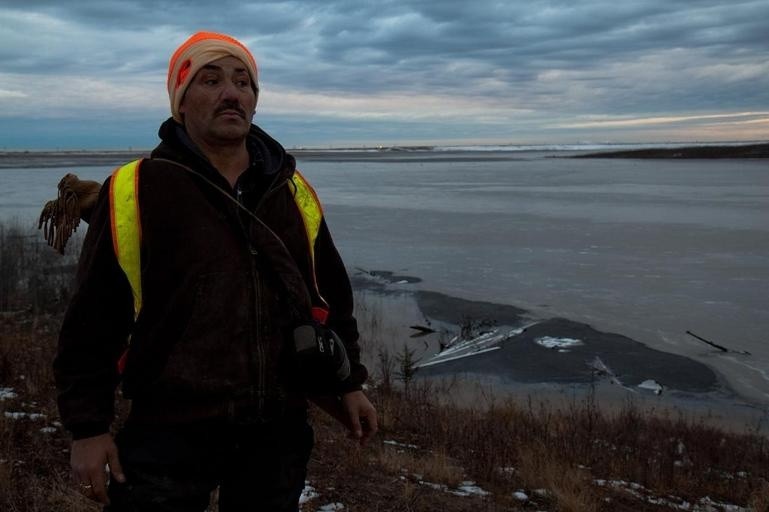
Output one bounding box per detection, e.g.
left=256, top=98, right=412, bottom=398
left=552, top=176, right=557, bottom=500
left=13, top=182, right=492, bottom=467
left=79, top=481, right=92, bottom=489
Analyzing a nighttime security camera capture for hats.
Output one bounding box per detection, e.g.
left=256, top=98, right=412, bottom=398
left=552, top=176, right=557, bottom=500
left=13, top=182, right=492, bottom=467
left=168, top=31, right=259, bottom=125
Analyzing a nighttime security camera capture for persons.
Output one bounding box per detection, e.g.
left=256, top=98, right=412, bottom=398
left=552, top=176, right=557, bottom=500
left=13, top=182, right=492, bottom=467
left=52, top=32, right=378, bottom=512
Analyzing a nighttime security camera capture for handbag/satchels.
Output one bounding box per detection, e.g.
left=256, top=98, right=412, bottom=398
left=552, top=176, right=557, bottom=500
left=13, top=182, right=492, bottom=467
left=284, top=317, right=350, bottom=390
